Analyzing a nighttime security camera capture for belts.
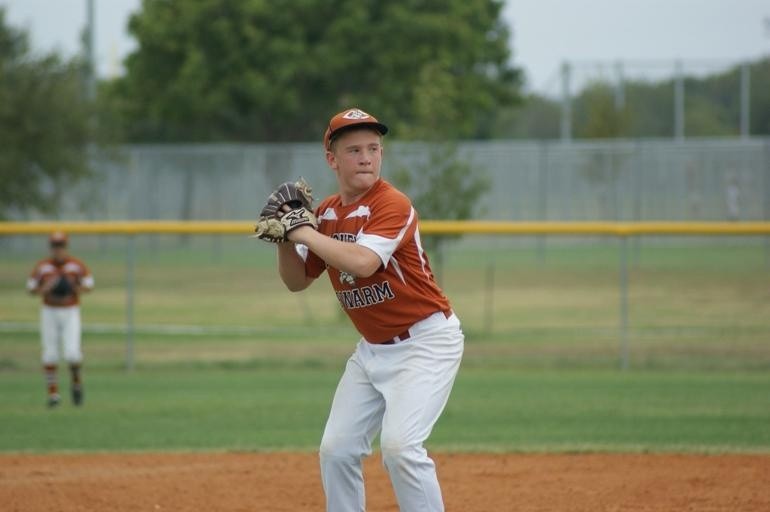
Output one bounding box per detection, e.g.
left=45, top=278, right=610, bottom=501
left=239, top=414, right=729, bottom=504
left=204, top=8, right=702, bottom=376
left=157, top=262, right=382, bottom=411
left=368, top=309, right=455, bottom=345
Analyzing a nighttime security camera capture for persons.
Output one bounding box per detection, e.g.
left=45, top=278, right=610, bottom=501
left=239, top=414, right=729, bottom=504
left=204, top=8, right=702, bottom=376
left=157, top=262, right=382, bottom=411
left=255, top=108, right=466, bottom=512
left=26, top=230, right=94, bottom=406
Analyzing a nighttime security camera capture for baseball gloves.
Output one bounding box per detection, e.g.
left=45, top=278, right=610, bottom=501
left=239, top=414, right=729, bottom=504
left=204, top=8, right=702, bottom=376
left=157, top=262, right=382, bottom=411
left=255, top=175, right=319, bottom=244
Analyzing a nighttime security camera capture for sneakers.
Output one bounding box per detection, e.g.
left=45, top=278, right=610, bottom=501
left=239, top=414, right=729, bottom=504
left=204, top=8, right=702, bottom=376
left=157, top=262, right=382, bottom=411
left=46, top=384, right=82, bottom=407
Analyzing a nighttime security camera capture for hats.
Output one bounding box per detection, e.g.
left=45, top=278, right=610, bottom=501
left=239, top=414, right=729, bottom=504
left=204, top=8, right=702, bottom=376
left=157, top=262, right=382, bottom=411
left=324, top=107, right=388, bottom=151
left=48, top=231, right=70, bottom=244
